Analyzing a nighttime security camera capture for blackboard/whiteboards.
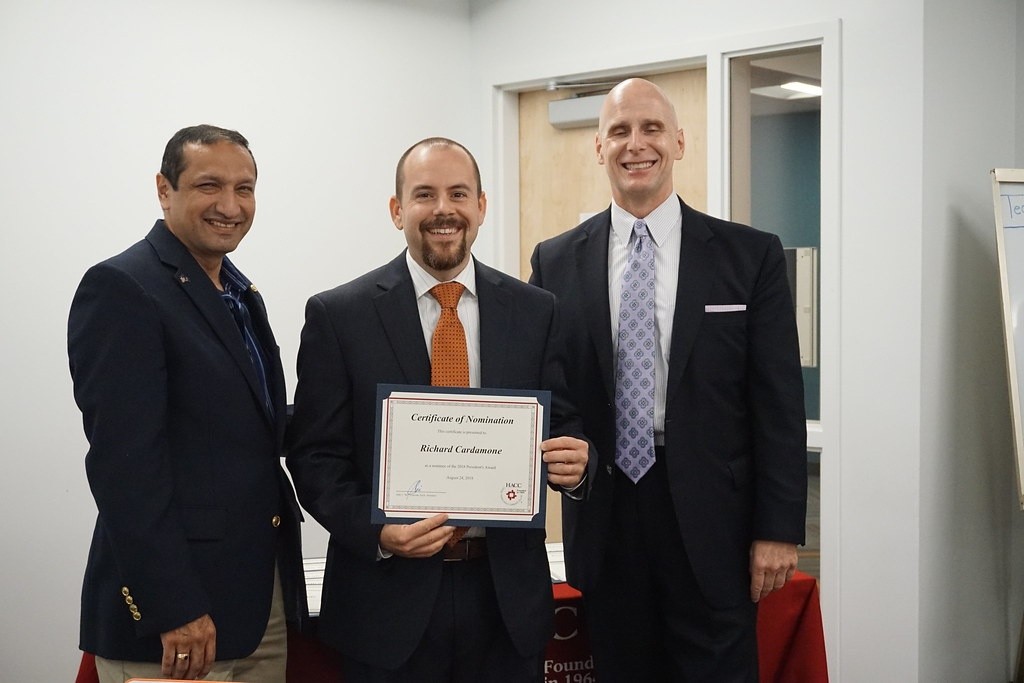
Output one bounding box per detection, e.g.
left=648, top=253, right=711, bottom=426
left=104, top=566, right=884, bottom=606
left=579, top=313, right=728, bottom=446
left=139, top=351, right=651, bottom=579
left=990, top=167, right=1024, bottom=514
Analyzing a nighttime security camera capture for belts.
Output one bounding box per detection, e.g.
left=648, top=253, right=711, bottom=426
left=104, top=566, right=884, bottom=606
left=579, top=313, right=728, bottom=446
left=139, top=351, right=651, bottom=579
left=442, top=538, right=485, bottom=563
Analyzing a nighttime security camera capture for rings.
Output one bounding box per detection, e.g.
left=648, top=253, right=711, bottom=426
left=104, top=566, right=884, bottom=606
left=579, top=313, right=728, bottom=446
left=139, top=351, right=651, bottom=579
left=177, top=653, right=189, bottom=661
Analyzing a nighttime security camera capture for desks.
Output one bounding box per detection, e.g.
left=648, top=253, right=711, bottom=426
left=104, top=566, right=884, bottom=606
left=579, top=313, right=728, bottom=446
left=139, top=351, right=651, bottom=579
left=73, top=542, right=829, bottom=683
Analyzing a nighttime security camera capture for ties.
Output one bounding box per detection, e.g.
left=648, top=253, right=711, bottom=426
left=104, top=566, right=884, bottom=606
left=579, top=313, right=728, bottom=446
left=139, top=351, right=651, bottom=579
left=615, top=219, right=657, bottom=485
left=430, top=283, right=473, bottom=549
left=220, top=283, right=278, bottom=418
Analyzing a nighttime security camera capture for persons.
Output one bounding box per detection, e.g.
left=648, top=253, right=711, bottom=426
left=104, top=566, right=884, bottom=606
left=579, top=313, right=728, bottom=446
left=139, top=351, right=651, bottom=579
left=68, top=124, right=312, bottom=683
left=529, top=78, right=809, bottom=683
left=286, top=138, right=600, bottom=683
